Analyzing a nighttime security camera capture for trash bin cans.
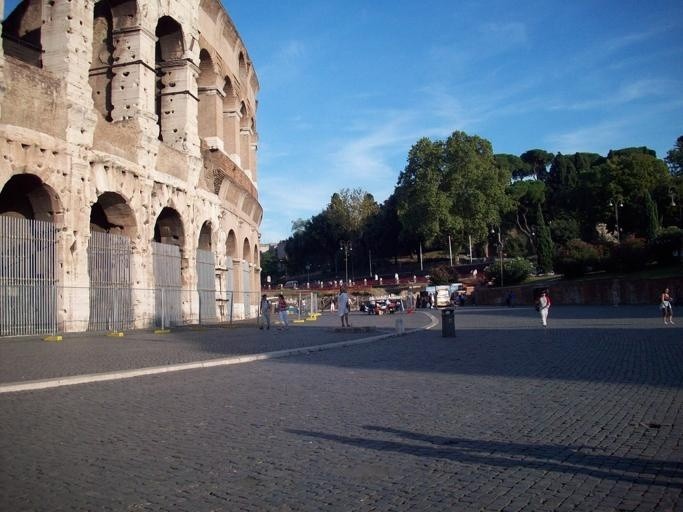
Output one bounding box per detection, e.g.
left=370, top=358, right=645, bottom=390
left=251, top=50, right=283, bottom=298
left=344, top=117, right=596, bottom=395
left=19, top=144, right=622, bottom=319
left=441, top=309, right=455, bottom=337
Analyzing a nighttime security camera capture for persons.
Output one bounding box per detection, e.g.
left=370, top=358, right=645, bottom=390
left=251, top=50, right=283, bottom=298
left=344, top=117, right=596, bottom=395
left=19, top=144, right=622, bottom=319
left=277, top=294, right=289, bottom=327
left=536, top=290, right=550, bottom=328
left=338, top=286, right=351, bottom=329
left=259, top=294, right=273, bottom=330
left=271, top=289, right=478, bottom=315
left=659, top=288, right=674, bottom=325
left=503, top=280, right=683, bottom=307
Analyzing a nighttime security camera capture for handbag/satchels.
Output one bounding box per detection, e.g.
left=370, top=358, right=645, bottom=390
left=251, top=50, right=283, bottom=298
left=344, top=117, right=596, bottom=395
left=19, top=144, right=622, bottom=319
left=280, top=306, right=289, bottom=311
left=534, top=300, right=542, bottom=312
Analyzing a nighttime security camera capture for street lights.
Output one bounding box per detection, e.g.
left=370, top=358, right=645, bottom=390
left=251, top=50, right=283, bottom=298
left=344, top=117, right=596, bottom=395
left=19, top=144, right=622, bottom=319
left=490, top=222, right=504, bottom=287
left=305, top=264, right=311, bottom=282
left=609, top=198, right=624, bottom=240
left=339, top=240, right=353, bottom=282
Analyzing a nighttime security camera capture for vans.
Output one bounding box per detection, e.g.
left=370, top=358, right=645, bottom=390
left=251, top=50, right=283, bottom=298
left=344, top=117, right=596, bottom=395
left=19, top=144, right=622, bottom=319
left=284, top=280, right=298, bottom=288
